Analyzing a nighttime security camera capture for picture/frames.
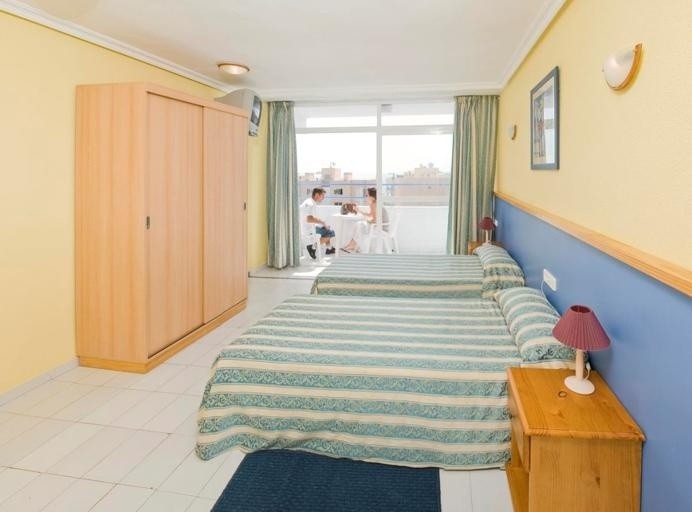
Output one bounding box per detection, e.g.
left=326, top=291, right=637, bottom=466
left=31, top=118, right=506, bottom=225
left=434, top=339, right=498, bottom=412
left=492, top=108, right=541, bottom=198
left=529, top=66, right=560, bottom=170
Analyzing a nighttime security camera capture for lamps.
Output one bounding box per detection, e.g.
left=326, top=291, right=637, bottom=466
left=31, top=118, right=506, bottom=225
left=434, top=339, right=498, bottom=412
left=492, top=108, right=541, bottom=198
left=217, top=63, right=250, bottom=74
left=552, top=304, right=611, bottom=397
left=506, top=124, right=516, bottom=140
left=601, top=33, right=643, bottom=91
left=478, top=217, right=496, bottom=246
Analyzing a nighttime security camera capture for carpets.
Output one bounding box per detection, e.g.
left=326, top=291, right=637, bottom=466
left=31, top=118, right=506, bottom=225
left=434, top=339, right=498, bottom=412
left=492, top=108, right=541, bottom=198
left=210, top=449, right=441, bottom=512
left=249, top=265, right=326, bottom=280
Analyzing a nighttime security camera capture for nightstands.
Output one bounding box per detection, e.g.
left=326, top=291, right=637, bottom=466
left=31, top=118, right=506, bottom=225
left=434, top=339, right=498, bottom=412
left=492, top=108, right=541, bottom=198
left=505, top=366, right=645, bottom=512
left=467, top=240, right=504, bottom=255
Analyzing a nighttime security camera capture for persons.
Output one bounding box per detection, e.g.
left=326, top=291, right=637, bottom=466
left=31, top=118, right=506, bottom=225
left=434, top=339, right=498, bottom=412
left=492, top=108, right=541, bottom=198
left=299, top=188, right=336, bottom=259
left=339, top=187, right=390, bottom=253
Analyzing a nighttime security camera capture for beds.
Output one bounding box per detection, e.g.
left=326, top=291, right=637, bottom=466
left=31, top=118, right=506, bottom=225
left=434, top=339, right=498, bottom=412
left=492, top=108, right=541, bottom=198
left=195, top=286, right=593, bottom=470
left=310, top=255, right=524, bottom=298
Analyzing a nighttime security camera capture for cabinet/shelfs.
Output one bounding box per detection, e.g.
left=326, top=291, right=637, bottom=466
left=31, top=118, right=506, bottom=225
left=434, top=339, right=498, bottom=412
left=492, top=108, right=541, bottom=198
left=73, top=82, right=249, bottom=374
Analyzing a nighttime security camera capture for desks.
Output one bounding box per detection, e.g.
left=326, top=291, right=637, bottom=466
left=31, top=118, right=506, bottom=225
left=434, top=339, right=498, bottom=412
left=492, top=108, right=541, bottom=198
left=333, top=213, right=362, bottom=257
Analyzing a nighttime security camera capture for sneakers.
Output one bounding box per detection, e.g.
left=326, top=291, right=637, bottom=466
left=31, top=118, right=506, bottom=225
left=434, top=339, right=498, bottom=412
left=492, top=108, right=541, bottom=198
left=340, top=247, right=353, bottom=253
left=326, top=248, right=335, bottom=254
left=307, top=245, right=316, bottom=258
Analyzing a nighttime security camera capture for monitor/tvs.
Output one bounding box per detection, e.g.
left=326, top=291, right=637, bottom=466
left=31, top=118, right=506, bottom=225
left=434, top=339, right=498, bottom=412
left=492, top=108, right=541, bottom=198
left=214, top=89, right=263, bottom=137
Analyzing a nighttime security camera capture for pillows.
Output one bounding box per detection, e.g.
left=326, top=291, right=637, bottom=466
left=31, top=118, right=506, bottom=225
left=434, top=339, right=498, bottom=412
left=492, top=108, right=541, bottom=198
left=494, top=287, right=589, bottom=363
left=473, top=244, right=524, bottom=290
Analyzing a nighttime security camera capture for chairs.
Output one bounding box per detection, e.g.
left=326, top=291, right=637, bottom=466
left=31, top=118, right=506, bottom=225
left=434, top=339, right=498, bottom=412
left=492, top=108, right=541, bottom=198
left=367, top=211, right=398, bottom=255
left=301, top=206, right=321, bottom=262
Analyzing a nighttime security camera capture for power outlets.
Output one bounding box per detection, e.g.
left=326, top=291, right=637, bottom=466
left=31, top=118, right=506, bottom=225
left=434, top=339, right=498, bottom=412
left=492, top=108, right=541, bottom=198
left=543, top=269, right=556, bottom=291
